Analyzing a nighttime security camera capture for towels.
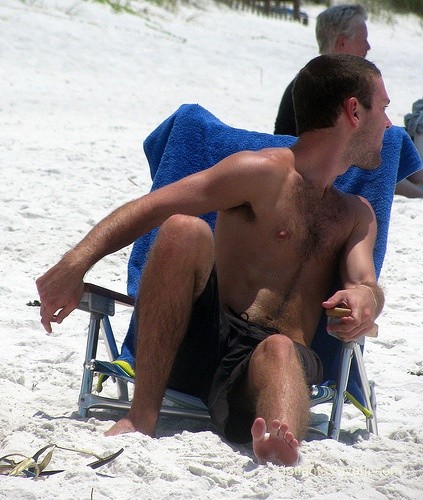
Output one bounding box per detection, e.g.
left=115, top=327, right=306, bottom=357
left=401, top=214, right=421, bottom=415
left=89, top=103, right=423, bottom=419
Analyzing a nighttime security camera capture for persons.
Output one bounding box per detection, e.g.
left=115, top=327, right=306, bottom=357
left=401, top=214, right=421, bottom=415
left=34, top=53, right=393, bottom=470
left=274, top=3, right=371, bottom=130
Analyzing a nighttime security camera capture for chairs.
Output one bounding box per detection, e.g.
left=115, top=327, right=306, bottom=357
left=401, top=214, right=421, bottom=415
left=76, top=102, right=423, bottom=445
